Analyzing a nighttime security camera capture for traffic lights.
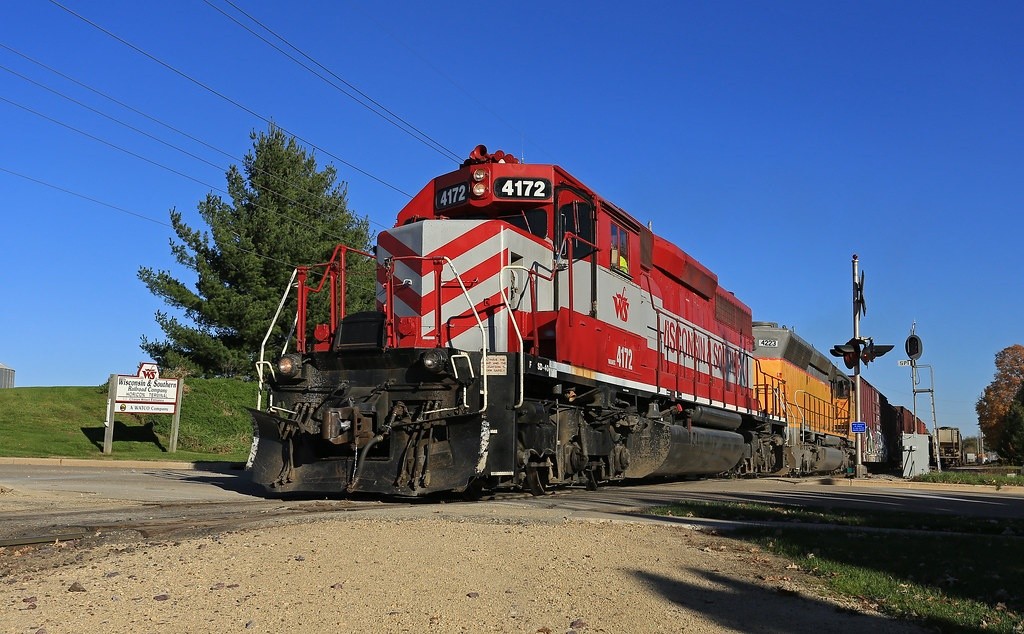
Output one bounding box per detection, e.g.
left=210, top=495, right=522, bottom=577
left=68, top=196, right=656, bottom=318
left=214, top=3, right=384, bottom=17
left=861, top=337, right=896, bottom=365
left=830, top=338, right=860, bottom=369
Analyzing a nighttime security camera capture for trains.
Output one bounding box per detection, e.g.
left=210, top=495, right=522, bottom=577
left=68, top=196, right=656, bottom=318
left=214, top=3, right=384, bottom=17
left=243, top=144, right=931, bottom=504
left=931, top=426, right=964, bottom=467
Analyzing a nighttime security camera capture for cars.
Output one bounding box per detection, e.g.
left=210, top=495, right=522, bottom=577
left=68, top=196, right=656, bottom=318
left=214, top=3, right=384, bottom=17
left=966, top=451, right=1003, bottom=466
